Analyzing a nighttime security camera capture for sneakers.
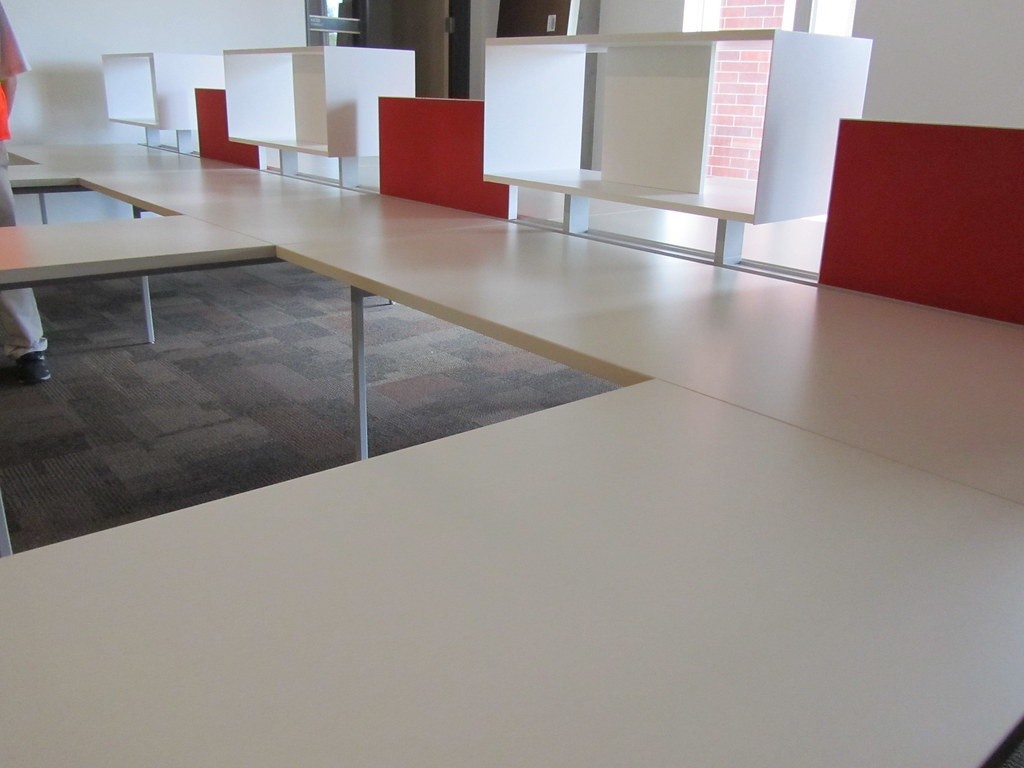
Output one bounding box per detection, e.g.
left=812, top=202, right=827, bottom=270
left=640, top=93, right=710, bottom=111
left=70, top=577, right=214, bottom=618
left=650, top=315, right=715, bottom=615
left=13, top=351, right=53, bottom=385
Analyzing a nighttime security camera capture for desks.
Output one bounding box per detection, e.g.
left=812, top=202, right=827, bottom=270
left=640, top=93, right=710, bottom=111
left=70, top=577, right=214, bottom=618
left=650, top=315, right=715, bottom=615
left=4, top=137, right=1024, bottom=768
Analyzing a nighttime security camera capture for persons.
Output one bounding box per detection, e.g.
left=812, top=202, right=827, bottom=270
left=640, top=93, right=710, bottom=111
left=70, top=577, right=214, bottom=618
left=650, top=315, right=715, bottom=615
left=0, top=1, right=54, bottom=388
